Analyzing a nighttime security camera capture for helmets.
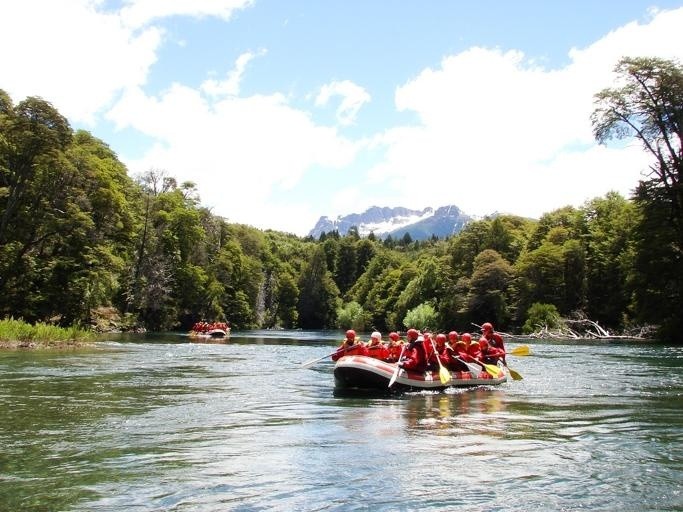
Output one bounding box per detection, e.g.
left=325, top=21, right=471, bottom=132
left=407, top=329, right=418, bottom=339
left=424, top=333, right=434, bottom=340
left=479, top=337, right=488, bottom=347
left=371, top=331, right=381, bottom=342
left=346, top=329, right=356, bottom=338
left=481, top=322, right=492, bottom=332
left=437, top=334, right=446, bottom=343
left=462, top=333, right=471, bottom=341
left=389, top=332, right=399, bottom=341
left=449, top=331, right=456, bottom=339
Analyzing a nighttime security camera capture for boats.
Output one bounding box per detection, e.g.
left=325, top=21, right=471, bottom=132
left=331, top=351, right=510, bottom=391
left=190, top=327, right=229, bottom=336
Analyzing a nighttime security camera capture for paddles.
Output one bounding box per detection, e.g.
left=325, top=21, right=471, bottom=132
left=303, top=344, right=359, bottom=368
left=430, top=338, right=450, bottom=384
left=470, top=355, right=499, bottom=377
left=387, top=346, right=406, bottom=388
left=452, top=354, right=482, bottom=376
left=499, top=358, right=523, bottom=379
left=506, top=345, right=532, bottom=356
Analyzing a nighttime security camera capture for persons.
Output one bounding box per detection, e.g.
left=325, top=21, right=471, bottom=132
left=330, top=321, right=506, bottom=373
left=191, top=320, right=228, bottom=332
